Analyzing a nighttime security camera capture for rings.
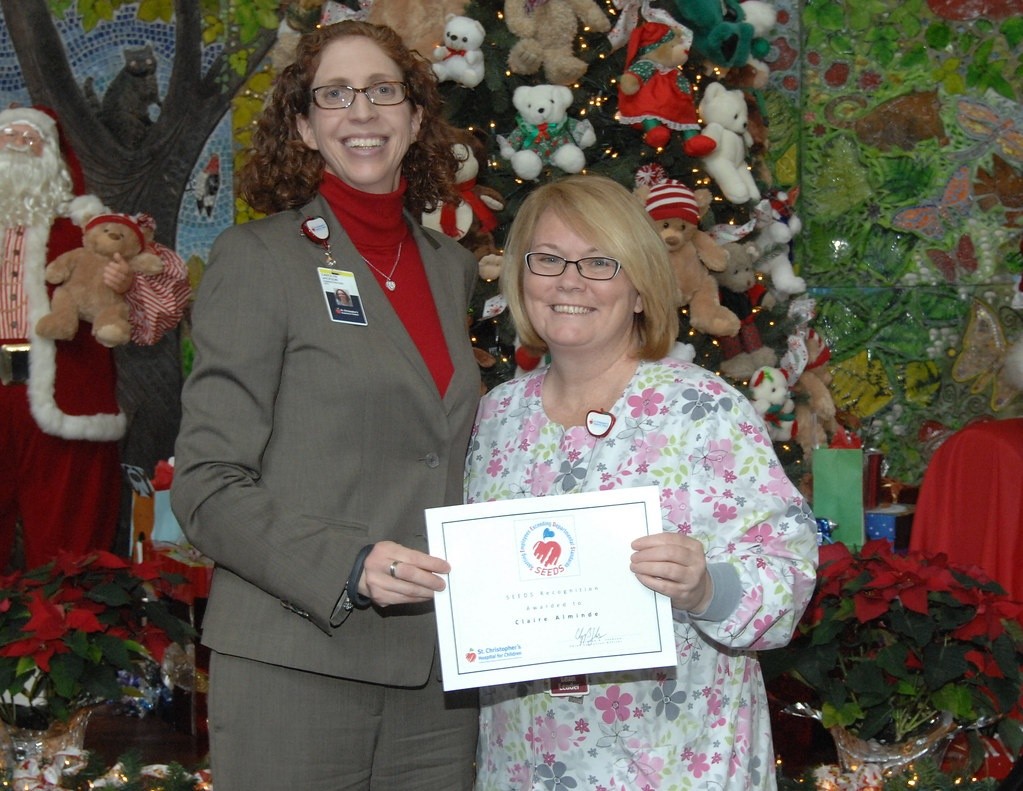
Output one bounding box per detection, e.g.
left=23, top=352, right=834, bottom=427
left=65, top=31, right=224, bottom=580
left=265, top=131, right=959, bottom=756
left=390, top=561, right=400, bottom=577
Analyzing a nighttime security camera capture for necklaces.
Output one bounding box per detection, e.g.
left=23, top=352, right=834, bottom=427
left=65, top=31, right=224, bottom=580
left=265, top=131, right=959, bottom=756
left=362, top=242, right=401, bottom=290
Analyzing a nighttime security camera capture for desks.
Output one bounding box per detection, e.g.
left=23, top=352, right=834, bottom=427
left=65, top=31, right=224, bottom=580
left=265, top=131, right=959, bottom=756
left=142, top=544, right=210, bottom=735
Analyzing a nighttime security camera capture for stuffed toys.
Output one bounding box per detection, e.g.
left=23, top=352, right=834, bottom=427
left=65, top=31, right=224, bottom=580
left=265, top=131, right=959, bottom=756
left=617, top=0, right=773, bottom=204
left=500, top=85, right=597, bottom=179
left=432, top=14, right=485, bottom=88
left=34, top=199, right=161, bottom=346
left=636, top=166, right=835, bottom=464
left=504, top=0, right=611, bottom=86
left=420, top=125, right=544, bottom=370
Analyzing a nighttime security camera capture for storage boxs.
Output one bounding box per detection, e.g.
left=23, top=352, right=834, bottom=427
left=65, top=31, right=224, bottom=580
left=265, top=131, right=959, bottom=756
left=865, top=503, right=916, bottom=554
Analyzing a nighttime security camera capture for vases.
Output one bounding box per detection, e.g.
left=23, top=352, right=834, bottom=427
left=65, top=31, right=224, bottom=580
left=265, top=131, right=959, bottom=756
left=830, top=722, right=959, bottom=782
left=0, top=708, right=91, bottom=762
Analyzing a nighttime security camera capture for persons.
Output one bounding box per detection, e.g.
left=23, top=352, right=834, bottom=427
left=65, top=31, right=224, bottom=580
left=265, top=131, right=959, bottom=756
left=463, top=177, right=819, bottom=791
left=336, top=289, right=351, bottom=306
left=169, top=21, right=481, bottom=790
left=0, top=103, right=134, bottom=726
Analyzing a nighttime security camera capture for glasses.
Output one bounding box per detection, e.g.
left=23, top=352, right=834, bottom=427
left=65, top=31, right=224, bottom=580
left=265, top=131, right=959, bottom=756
left=311, top=81, right=412, bottom=109
left=525, top=252, right=622, bottom=280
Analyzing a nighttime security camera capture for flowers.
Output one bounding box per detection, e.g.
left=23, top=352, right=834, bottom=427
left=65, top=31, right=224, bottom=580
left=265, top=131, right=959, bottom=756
left=774, top=536, right=1023, bottom=791
left=0, top=544, right=202, bottom=736
